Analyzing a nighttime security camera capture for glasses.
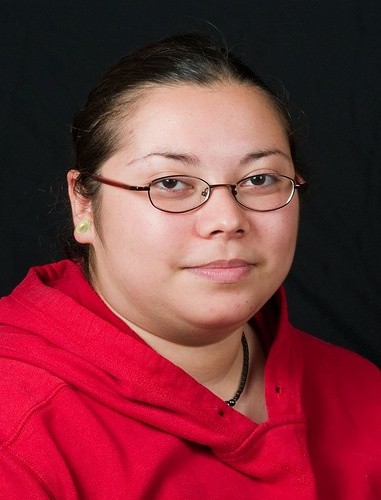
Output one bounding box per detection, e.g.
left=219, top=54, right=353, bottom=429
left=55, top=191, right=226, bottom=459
left=86, top=171, right=307, bottom=215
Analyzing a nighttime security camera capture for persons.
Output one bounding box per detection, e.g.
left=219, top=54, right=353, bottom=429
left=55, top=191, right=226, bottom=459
left=0, top=34, right=381, bottom=500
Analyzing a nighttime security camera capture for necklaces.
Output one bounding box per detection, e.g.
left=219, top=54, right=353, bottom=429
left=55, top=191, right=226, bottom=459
left=225, top=331, right=249, bottom=406
